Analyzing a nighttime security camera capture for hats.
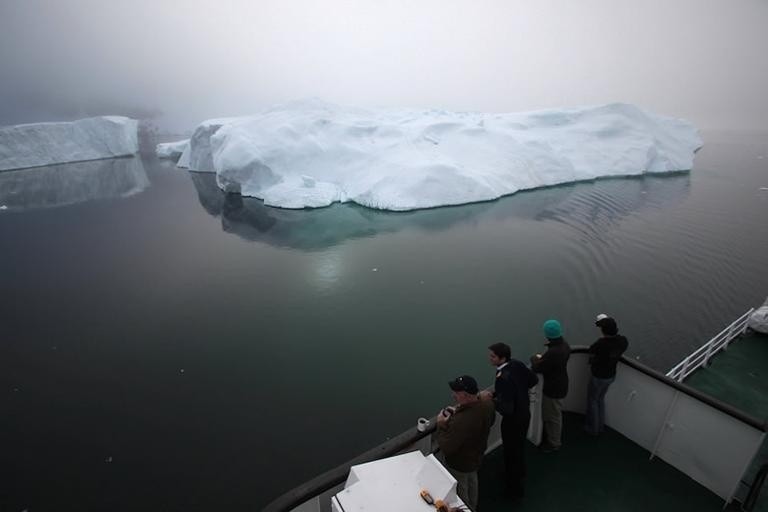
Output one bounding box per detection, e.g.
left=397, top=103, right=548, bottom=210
left=543, top=320, right=561, bottom=339
left=595, top=317, right=616, bottom=331
left=448, top=375, right=478, bottom=394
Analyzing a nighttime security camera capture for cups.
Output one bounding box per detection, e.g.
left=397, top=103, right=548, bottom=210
left=417, top=418, right=430, bottom=432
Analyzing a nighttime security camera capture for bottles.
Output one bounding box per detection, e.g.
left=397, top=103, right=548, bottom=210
left=536, top=352, right=542, bottom=359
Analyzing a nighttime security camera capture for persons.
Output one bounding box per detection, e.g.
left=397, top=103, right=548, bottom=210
left=580, top=317, right=627, bottom=436
left=532, top=320, right=572, bottom=453
left=436, top=374, right=496, bottom=512
left=480, top=341, right=538, bottom=479
